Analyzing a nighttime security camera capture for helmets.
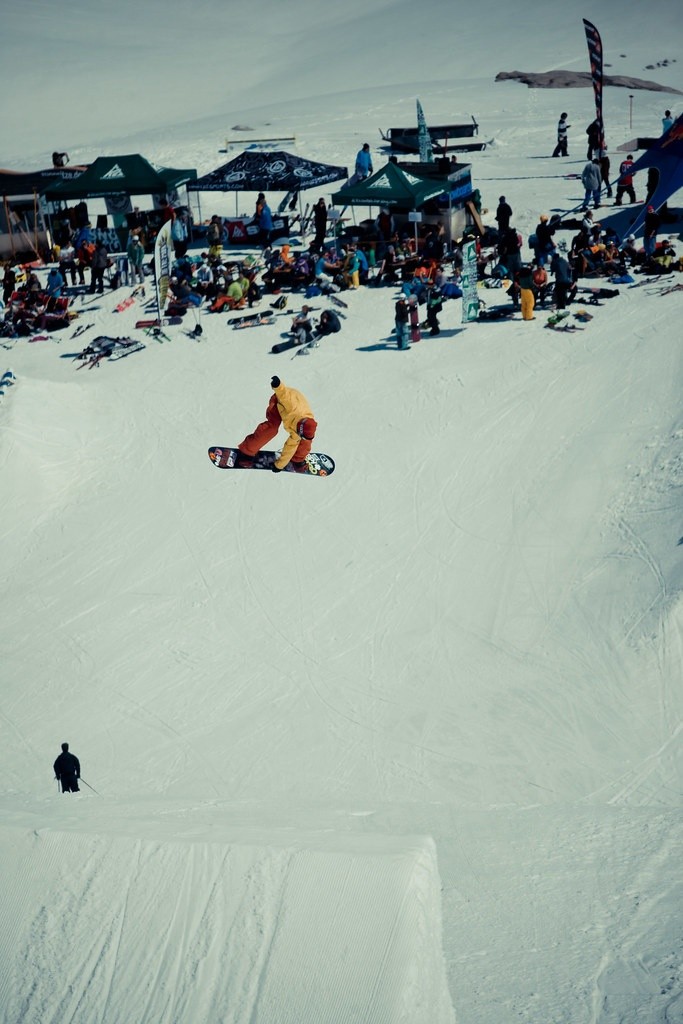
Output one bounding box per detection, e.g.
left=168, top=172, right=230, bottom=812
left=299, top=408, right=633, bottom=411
left=132, top=235, right=140, bottom=241
left=540, top=215, right=548, bottom=222
left=399, top=292, right=406, bottom=300
left=628, top=233, right=635, bottom=241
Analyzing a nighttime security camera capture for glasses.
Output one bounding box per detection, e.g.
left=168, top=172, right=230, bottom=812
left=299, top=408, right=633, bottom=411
left=301, top=435, right=314, bottom=441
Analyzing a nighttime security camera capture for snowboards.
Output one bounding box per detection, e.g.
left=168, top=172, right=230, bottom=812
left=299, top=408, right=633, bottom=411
left=208, top=446, right=337, bottom=477
left=577, top=286, right=606, bottom=293
left=228, top=310, right=277, bottom=330
left=548, top=311, right=570, bottom=324
left=136, top=317, right=182, bottom=329
left=408, top=293, right=421, bottom=342
left=116, top=296, right=135, bottom=313
left=271, top=331, right=323, bottom=354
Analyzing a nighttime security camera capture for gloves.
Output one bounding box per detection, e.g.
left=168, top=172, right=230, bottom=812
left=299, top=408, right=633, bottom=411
left=269, top=462, right=281, bottom=473
left=271, top=376, right=280, bottom=388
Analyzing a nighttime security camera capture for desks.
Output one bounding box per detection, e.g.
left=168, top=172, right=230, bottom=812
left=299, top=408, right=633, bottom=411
left=218, top=214, right=289, bottom=247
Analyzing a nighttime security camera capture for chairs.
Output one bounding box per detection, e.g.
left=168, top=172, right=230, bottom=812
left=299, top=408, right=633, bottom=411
left=7, top=290, right=71, bottom=328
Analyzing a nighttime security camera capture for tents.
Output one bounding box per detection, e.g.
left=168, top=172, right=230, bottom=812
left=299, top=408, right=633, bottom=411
left=187, top=150, right=356, bottom=248
left=1, top=154, right=202, bottom=251
left=332, top=163, right=451, bottom=255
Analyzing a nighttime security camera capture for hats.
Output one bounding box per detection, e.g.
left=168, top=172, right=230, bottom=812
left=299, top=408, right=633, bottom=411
left=297, top=418, right=317, bottom=439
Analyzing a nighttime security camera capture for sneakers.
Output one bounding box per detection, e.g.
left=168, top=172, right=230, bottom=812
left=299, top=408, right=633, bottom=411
left=238, top=449, right=255, bottom=468
left=292, top=459, right=307, bottom=472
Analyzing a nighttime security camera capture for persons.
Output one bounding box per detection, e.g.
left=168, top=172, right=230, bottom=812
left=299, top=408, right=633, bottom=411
left=355, top=143, right=373, bottom=180
left=661, top=110, right=674, bottom=131
left=586, top=120, right=598, bottom=160
left=238, top=375, right=318, bottom=474
left=0, top=227, right=145, bottom=299
left=52, top=152, right=70, bottom=168
left=553, top=113, right=571, bottom=157
left=613, top=154, right=636, bottom=204
left=495, top=196, right=676, bottom=320
left=600, top=151, right=613, bottom=198
left=54, top=743, right=81, bottom=793
left=167, top=191, right=486, bottom=350
left=581, top=159, right=601, bottom=211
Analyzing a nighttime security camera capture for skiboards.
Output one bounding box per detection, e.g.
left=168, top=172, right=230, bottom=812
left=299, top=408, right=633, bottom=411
left=109, top=342, right=145, bottom=360
